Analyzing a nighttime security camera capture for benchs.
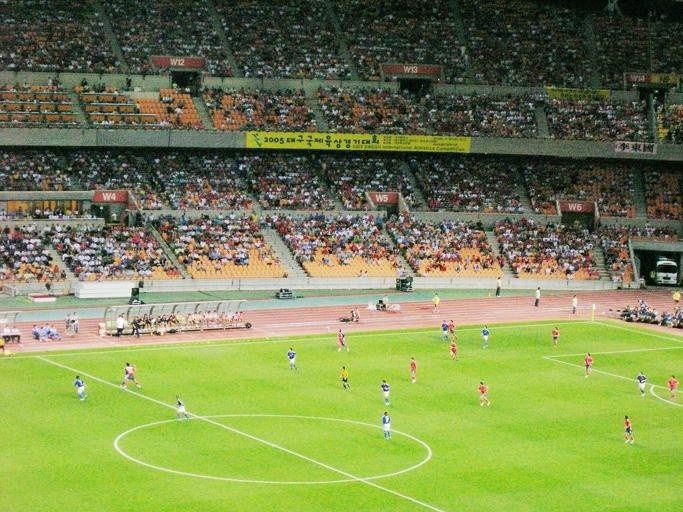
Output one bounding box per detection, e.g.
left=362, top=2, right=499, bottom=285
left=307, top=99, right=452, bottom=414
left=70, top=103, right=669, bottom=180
left=104, top=320, right=246, bottom=336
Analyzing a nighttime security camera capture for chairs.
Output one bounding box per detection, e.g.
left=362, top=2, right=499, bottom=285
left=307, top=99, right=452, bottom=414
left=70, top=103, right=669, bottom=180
left=0, top=0, right=682, bottom=295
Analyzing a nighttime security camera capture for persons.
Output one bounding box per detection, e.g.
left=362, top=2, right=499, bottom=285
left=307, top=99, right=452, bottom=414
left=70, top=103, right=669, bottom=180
left=340, top=355, right=416, bottom=440
left=31, top=311, right=79, bottom=342
left=337, top=329, right=349, bottom=353
left=0, top=324, right=21, bottom=353
left=619, top=290, right=683, bottom=328
left=572, top=295, right=577, bottom=314
left=114, top=310, right=243, bottom=338
left=477, top=382, right=491, bottom=406
left=383, top=294, right=390, bottom=311
left=287, top=348, right=297, bottom=370
left=496, top=276, right=501, bottom=297
left=1, top=1, right=683, bottom=143
left=440, top=320, right=490, bottom=359
left=73, top=375, right=87, bottom=400
left=176, top=394, right=190, bottom=422
left=0, top=144, right=683, bottom=289
left=535, top=287, right=541, bottom=307
left=584, top=353, right=680, bottom=445
left=120, top=362, right=141, bottom=389
left=432, top=293, right=440, bottom=313
left=552, top=327, right=559, bottom=348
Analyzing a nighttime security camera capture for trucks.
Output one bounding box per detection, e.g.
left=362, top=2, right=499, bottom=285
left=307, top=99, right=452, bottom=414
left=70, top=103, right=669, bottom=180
left=654, top=256, right=678, bottom=286
